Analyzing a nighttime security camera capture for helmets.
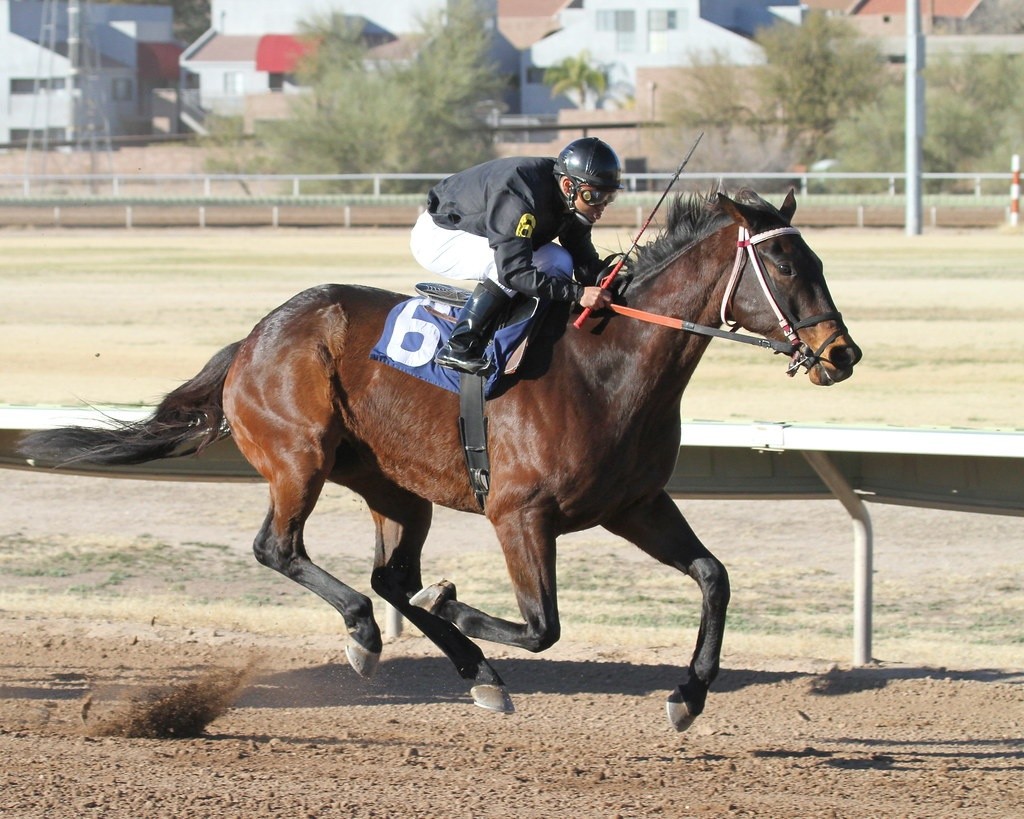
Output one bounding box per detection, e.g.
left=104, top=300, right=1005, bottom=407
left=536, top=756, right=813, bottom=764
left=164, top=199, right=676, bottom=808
left=553, top=137, right=625, bottom=189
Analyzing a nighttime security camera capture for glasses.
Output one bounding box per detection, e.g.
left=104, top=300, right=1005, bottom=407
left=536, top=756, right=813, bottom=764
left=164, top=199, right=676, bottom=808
left=579, top=190, right=618, bottom=206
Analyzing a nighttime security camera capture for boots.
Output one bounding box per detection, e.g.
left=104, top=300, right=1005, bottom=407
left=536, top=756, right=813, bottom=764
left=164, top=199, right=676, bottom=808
left=434, top=282, right=508, bottom=375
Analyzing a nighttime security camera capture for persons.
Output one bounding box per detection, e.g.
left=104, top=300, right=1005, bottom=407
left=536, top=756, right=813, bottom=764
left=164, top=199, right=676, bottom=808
left=410, top=137, right=622, bottom=375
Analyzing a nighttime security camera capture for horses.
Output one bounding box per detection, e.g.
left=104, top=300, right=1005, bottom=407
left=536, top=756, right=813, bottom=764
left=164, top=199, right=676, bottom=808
left=0, top=185, right=864, bottom=734
